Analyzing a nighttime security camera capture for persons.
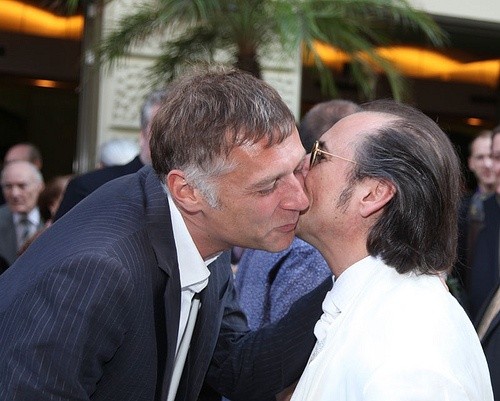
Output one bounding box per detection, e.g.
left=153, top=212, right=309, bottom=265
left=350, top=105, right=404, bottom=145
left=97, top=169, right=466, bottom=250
left=293, top=98, right=496, bottom=401
left=456, top=128, right=495, bottom=294
left=468, top=125, right=500, bottom=317
left=0, top=63, right=337, bottom=401
left=220, top=98, right=364, bottom=401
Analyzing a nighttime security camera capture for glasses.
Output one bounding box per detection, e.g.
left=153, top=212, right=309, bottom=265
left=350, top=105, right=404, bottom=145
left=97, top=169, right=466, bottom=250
left=310, top=141, right=360, bottom=169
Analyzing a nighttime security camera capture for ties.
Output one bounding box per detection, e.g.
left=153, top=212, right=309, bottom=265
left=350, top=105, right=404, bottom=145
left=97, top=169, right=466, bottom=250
left=166, top=291, right=202, bottom=401
left=20, top=215, right=32, bottom=238
